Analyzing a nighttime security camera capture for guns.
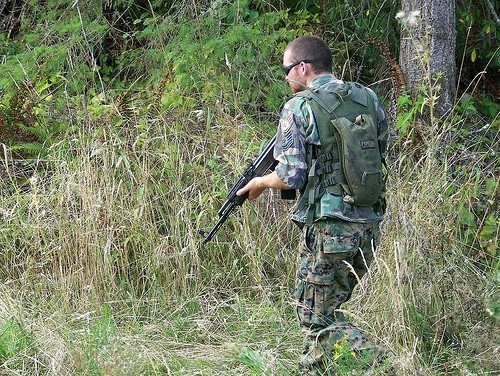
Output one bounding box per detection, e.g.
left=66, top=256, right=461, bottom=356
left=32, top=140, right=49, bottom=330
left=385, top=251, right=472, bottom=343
left=200, top=121, right=298, bottom=245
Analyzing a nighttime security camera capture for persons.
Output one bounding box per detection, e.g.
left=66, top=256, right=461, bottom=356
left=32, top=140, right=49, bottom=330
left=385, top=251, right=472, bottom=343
left=236, top=35, right=391, bottom=376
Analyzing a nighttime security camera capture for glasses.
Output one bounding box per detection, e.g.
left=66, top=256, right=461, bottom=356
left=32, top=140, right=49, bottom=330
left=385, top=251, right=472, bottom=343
left=283, top=59, right=311, bottom=76
